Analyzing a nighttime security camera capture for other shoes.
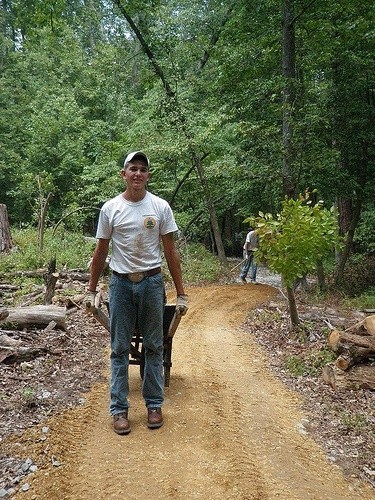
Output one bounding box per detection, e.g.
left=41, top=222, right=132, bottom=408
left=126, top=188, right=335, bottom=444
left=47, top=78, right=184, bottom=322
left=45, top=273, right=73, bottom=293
left=251, top=280, right=258, bottom=284
left=241, top=279, right=247, bottom=284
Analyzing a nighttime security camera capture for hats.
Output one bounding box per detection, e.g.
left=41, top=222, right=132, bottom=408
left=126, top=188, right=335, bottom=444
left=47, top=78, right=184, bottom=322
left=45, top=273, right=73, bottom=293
left=124, top=151, right=149, bottom=169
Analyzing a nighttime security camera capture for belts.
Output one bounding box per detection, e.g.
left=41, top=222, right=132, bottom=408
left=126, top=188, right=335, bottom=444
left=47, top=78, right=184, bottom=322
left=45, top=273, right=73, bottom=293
left=112, top=266, right=161, bottom=283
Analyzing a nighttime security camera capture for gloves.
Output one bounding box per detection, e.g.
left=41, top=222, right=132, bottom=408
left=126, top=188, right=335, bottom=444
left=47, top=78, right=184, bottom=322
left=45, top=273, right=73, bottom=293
left=83, top=289, right=98, bottom=312
left=175, top=294, right=189, bottom=316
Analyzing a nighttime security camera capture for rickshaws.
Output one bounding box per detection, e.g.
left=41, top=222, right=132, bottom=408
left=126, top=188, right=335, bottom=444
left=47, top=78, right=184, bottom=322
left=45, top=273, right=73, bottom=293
left=85, top=295, right=183, bottom=387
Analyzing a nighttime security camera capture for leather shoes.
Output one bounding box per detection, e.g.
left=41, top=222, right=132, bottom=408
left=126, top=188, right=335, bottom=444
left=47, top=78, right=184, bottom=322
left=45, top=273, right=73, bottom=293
left=148, top=407, right=163, bottom=427
left=113, top=413, right=131, bottom=434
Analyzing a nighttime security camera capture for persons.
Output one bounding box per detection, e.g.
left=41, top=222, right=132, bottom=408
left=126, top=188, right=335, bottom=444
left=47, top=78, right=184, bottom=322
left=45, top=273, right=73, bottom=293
left=238, top=229, right=258, bottom=285
left=84, top=151, right=189, bottom=435
